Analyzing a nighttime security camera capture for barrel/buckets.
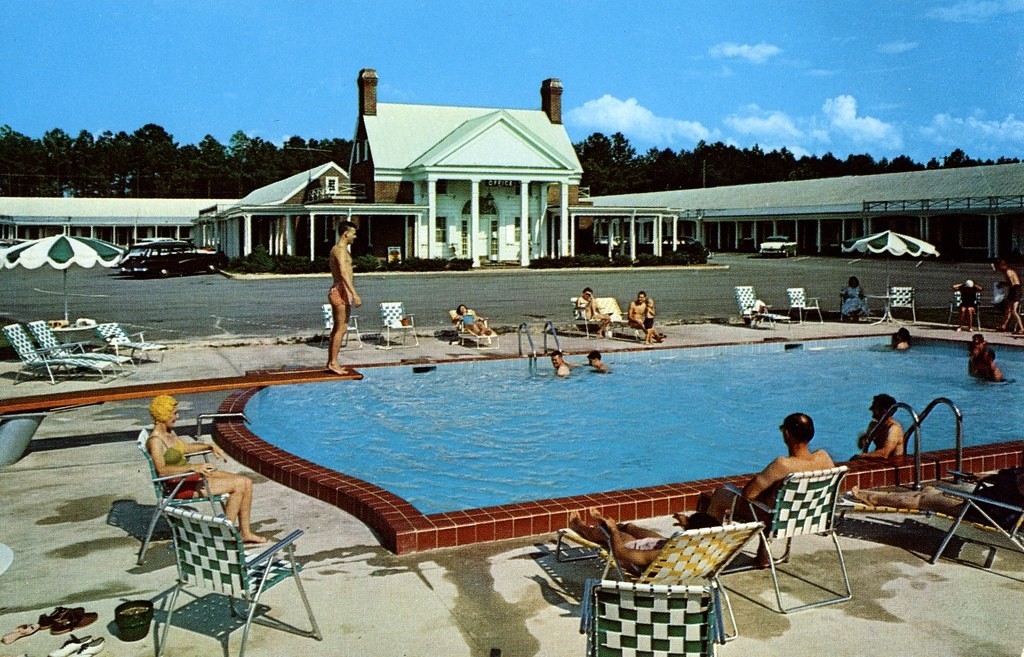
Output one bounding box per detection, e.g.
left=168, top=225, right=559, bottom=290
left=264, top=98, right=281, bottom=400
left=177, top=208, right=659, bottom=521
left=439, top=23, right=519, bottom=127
left=115, top=600, right=154, bottom=642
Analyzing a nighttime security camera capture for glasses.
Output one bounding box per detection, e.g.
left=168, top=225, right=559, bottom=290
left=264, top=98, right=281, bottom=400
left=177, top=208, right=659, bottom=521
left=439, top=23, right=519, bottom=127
left=779, top=425, right=786, bottom=431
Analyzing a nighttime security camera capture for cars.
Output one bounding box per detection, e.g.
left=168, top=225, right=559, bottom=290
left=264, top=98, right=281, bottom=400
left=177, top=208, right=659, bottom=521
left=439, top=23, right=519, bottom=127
left=661, top=235, right=711, bottom=259
left=595, top=235, right=628, bottom=245
left=117, top=235, right=227, bottom=279
left=757, top=234, right=798, bottom=259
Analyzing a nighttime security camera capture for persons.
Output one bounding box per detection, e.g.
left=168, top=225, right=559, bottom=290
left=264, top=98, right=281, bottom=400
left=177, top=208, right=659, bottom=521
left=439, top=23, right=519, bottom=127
left=628, top=290, right=668, bottom=343
left=967, top=334, right=1002, bottom=382
left=587, top=350, right=609, bottom=373
left=568, top=507, right=716, bottom=570
left=550, top=351, right=571, bottom=376
left=674, top=411, right=836, bottom=529
left=576, top=287, right=610, bottom=338
left=851, top=464, right=1024, bottom=533
left=991, top=258, right=1024, bottom=334
left=950, top=279, right=985, bottom=331
left=895, top=328, right=911, bottom=349
left=644, top=297, right=656, bottom=346
left=326, top=220, right=363, bottom=376
left=144, top=395, right=268, bottom=543
left=848, top=394, right=904, bottom=459
left=840, top=276, right=868, bottom=320
left=452, top=304, right=493, bottom=337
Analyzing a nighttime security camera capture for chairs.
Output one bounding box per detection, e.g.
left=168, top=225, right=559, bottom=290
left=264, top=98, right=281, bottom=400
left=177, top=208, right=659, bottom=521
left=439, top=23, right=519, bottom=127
left=95, top=322, right=168, bottom=368
left=157, top=506, right=323, bottom=657
left=948, top=288, right=1024, bottom=333
left=840, top=286, right=916, bottom=323
left=376, top=302, right=419, bottom=350
left=735, top=285, right=791, bottom=330
left=595, top=297, right=643, bottom=342
left=932, top=479, right=1024, bottom=564
left=786, top=288, right=823, bottom=324
left=27, top=320, right=137, bottom=377
left=552, top=297, right=616, bottom=339
left=2, top=323, right=117, bottom=383
left=320, top=304, right=362, bottom=351
left=557, top=465, right=852, bottom=657
left=449, top=309, right=500, bottom=349
left=137, top=428, right=231, bottom=563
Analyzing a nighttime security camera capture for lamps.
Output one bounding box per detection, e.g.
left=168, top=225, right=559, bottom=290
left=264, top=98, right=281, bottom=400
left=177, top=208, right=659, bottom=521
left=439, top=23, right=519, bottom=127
left=485, top=187, right=495, bottom=208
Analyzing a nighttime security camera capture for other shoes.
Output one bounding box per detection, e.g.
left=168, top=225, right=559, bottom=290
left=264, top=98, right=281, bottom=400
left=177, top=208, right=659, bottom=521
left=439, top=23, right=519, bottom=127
left=996, top=325, right=1005, bottom=332
left=48, top=634, right=93, bottom=657
left=50, top=611, right=98, bottom=635
left=37, top=606, right=85, bottom=630
left=1012, top=331, right=1024, bottom=335
left=68, top=637, right=105, bottom=657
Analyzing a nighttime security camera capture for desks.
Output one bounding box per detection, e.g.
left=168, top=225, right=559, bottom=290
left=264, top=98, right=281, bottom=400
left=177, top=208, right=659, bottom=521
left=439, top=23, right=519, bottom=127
left=866, top=294, right=911, bottom=326
left=49, top=323, right=99, bottom=352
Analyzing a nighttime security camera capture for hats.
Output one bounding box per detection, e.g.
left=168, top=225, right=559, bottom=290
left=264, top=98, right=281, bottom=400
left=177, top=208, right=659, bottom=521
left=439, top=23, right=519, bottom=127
left=965, top=279, right=974, bottom=288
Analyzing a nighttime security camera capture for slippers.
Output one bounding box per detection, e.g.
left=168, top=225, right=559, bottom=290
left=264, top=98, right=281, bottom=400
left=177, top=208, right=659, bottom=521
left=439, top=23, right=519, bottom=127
left=1, top=623, right=40, bottom=645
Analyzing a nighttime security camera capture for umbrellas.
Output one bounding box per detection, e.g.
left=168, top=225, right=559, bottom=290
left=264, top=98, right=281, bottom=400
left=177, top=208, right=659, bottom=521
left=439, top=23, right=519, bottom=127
left=0, top=234, right=127, bottom=363
left=841, top=229, right=940, bottom=325
